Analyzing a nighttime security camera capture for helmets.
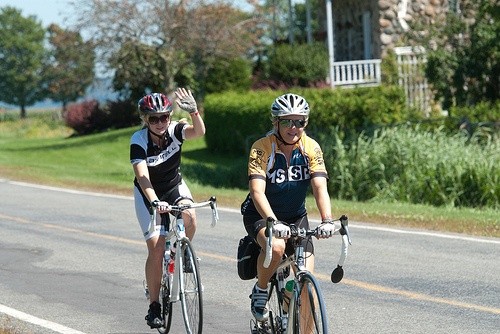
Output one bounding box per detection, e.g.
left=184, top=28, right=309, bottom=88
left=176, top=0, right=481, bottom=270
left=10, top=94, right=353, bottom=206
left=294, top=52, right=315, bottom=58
left=138, top=93, right=174, bottom=116
left=270, top=93, right=311, bottom=119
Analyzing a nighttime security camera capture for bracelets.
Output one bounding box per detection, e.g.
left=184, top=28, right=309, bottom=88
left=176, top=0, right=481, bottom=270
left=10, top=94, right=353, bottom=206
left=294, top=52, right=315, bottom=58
left=190, top=112, right=198, bottom=118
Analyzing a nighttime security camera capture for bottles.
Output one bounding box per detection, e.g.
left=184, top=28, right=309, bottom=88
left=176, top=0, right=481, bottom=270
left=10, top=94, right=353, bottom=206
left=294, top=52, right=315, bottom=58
left=165, top=241, right=172, bottom=271
left=281, top=280, right=294, bottom=313
left=168, top=242, right=178, bottom=261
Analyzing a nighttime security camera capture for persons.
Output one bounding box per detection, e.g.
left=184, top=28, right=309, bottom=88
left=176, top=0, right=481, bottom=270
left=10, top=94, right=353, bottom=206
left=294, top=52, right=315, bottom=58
left=241, top=94, right=335, bottom=334
left=129, top=88, right=208, bottom=328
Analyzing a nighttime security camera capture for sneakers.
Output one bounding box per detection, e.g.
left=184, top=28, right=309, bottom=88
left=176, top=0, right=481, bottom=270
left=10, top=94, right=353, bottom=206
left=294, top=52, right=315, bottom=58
left=182, top=245, right=200, bottom=273
left=147, top=301, right=164, bottom=328
left=250, top=281, right=270, bottom=322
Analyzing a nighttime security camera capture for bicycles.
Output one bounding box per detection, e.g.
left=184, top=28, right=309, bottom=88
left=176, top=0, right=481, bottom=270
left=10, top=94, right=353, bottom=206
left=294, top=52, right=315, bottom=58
left=250, top=214, right=351, bottom=332
left=142, top=195, right=220, bottom=334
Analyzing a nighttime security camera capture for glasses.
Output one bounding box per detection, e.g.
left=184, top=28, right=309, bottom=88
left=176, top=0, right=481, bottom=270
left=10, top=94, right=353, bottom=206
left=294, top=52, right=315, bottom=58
left=144, top=114, right=170, bottom=125
left=278, top=117, right=306, bottom=128
